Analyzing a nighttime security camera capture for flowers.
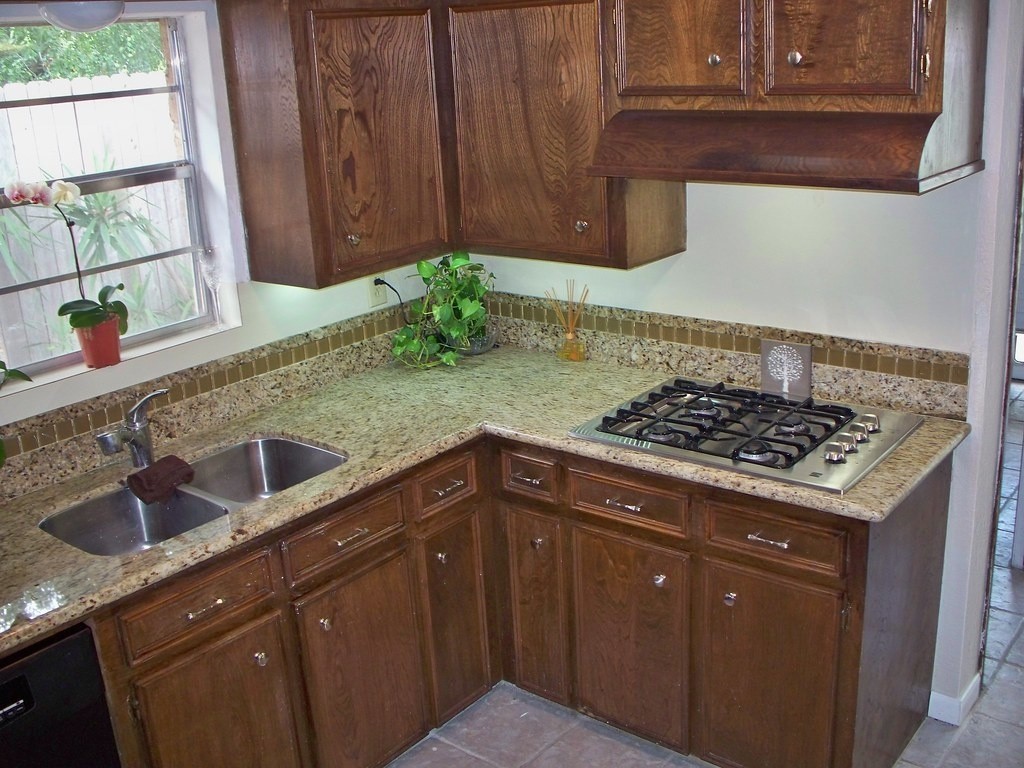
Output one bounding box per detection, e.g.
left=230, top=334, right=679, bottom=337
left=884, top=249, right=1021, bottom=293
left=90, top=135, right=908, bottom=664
left=4, top=180, right=128, bottom=335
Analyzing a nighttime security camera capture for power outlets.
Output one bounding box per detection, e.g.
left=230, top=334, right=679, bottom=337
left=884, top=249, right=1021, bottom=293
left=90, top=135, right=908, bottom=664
left=368, top=272, right=388, bottom=307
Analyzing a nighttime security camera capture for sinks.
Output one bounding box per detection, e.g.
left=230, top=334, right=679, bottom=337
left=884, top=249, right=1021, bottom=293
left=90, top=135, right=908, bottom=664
left=172, top=424, right=352, bottom=506
left=29, top=469, right=229, bottom=558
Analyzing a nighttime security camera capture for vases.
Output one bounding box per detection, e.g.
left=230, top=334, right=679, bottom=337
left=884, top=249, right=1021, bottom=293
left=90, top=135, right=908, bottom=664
left=76, top=313, right=121, bottom=368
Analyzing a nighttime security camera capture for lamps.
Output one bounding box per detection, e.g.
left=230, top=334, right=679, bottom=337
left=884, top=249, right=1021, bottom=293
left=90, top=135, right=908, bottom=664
left=38, top=0, right=124, bottom=32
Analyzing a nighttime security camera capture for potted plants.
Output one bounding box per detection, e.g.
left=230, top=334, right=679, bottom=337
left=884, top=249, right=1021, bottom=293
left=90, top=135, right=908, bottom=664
left=389, top=250, right=497, bottom=371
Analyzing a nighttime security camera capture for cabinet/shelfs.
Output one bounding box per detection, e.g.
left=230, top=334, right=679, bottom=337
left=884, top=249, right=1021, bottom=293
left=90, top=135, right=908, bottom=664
left=441, top=0, right=687, bottom=269
left=566, top=453, right=954, bottom=768
left=403, top=434, right=505, bottom=728
left=587, top=0, right=989, bottom=195
left=216, top=0, right=457, bottom=291
left=487, top=433, right=574, bottom=708
left=93, top=475, right=431, bottom=768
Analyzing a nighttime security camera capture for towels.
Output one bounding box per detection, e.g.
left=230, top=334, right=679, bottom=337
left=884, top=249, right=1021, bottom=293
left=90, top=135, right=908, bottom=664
left=126, top=454, right=197, bottom=506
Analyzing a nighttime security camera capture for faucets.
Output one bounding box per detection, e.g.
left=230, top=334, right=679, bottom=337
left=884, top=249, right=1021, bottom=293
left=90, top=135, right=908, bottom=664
left=92, top=386, right=173, bottom=470
